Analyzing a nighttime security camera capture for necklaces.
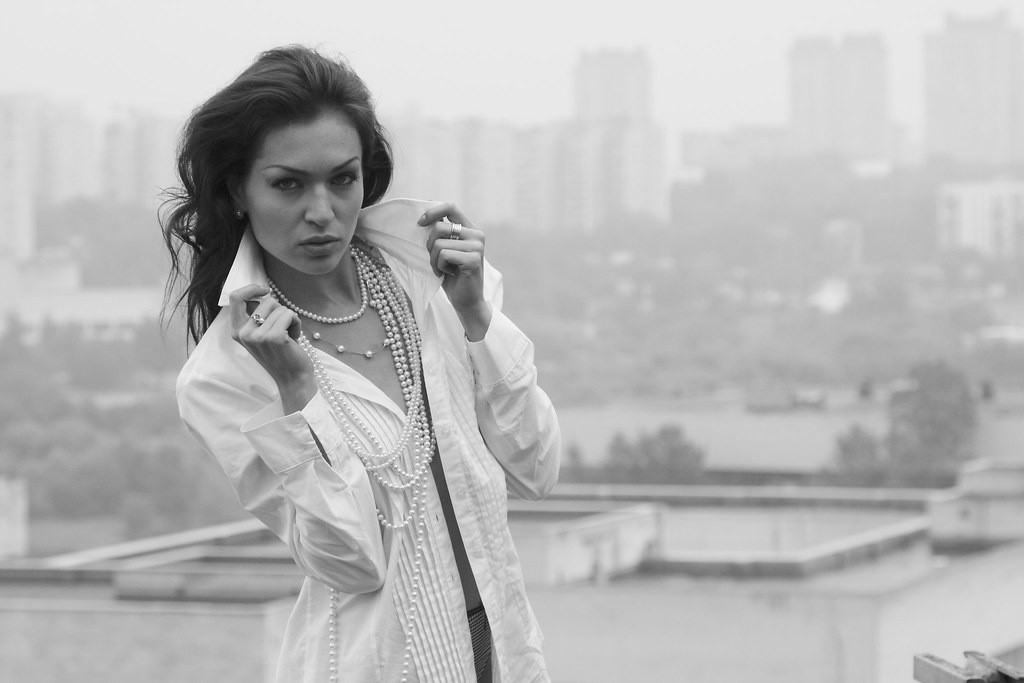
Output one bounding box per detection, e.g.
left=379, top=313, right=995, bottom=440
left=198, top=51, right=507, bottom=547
left=267, top=240, right=438, bottom=683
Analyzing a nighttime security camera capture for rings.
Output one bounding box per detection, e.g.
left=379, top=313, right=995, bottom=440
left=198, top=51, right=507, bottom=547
left=251, top=313, right=265, bottom=325
left=450, top=222, right=462, bottom=240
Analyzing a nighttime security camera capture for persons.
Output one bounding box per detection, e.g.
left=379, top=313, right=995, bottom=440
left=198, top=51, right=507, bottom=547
left=158, top=44, right=563, bottom=683
